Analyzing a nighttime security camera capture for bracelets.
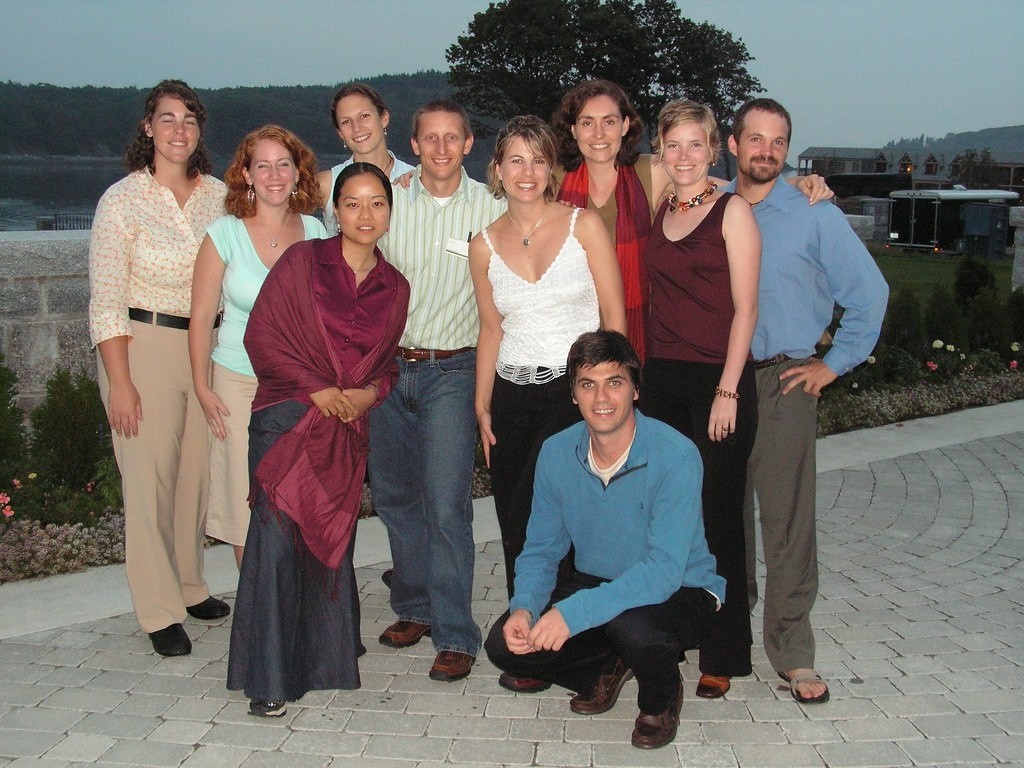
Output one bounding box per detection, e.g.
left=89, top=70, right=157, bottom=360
left=714, top=386, right=738, bottom=399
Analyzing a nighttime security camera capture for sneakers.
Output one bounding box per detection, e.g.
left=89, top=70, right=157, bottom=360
left=428, top=651, right=476, bottom=682
left=379, top=619, right=431, bottom=649
left=569, top=658, right=634, bottom=716
left=499, top=672, right=552, bottom=693
left=631, top=681, right=683, bottom=750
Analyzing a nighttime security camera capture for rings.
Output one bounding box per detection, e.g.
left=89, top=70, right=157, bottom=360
left=723, top=426, right=729, bottom=431
left=825, top=186, right=830, bottom=191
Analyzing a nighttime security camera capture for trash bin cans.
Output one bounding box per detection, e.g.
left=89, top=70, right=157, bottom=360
left=962, top=202, right=1010, bottom=255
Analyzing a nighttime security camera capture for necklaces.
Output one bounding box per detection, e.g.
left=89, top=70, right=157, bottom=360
left=267, top=233, right=280, bottom=247
left=506, top=208, right=543, bottom=246
left=384, top=158, right=391, bottom=173
left=667, top=180, right=718, bottom=212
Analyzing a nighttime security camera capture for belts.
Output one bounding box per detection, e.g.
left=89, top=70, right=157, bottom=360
left=754, top=354, right=789, bottom=370
left=396, top=346, right=473, bottom=363
left=130, top=307, right=223, bottom=330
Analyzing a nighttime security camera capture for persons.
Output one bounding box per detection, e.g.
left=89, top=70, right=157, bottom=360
left=465, top=112, right=633, bottom=694
left=86, top=79, right=233, bottom=656
left=391, top=81, right=836, bottom=382
left=638, top=97, right=756, bottom=700
left=367, top=99, right=577, bottom=682
left=186, top=122, right=329, bottom=573
left=303, top=78, right=414, bottom=238
left=482, top=329, right=726, bottom=752
left=220, top=159, right=411, bottom=717
left=717, top=96, right=890, bottom=705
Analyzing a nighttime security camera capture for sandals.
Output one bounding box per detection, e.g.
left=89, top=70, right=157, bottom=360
left=779, top=668, right=830, bottom=703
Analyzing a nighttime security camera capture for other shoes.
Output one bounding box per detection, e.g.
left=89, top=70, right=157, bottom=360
left=381, top=570, right=394, bottom=590
left=187, top=596, right=230, bottom=620
left=695, top=673, right=730, bottom=699
left=148, top=623, right=192, bottom=656
left=250, top=699, right=288, bottom=716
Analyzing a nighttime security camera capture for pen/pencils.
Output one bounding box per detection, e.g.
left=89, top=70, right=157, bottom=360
left=468, top=232, right=472, bottom=242
